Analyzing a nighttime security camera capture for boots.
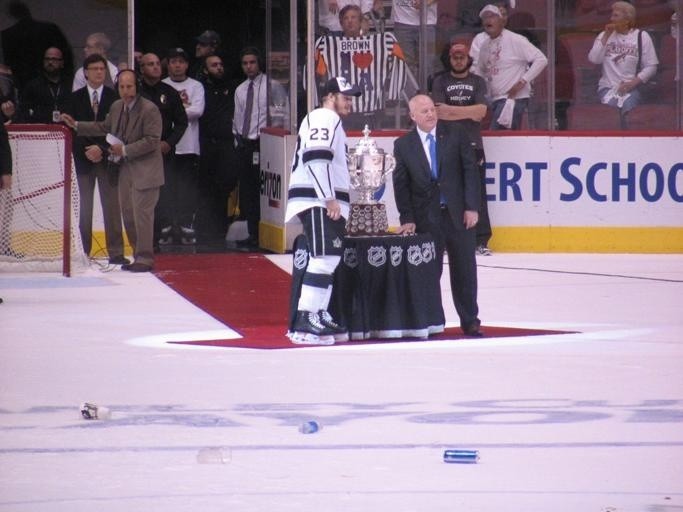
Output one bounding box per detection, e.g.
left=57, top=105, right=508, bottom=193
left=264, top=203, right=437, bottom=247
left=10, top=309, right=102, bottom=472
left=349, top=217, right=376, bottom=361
left=291, top=310, right=348, bottom=335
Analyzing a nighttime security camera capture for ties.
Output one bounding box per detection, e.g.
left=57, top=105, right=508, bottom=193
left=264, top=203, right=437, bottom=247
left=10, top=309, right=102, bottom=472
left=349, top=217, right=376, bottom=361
left=91, top=90, right=99, bottom=115
left=241, top=81, right=254, bottom=141
left=426, top=133, right=446, bottom=203
left=121, top=106, right=129, bottom=135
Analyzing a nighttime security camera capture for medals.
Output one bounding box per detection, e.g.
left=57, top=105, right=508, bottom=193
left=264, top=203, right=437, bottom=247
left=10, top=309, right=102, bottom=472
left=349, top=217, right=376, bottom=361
left=345, top=123, right=397, bottom=205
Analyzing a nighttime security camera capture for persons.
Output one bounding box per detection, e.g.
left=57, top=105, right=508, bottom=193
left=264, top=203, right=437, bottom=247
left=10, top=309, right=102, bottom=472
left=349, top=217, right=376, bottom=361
left=61, top=68, right=162, bottom=271
left=373, top=0, right=438, bottom=90
left=467, top=4, right=548, bottom=132
left=316, top=0, right=375, bottom=38
left=283, top=77, right=362, bottom=338
left=2, top=2, right=287, bottom=256
left=588, top=1, right=660, bottom=115
left=431, top=44, right=492, bottom=255
left=392, top=93, right=482, bottom=337
left=61, top=53, right=132, bottom=264
left=339, top=5, right=364, bottom=37
left=494, top=3, right=510, bottom=27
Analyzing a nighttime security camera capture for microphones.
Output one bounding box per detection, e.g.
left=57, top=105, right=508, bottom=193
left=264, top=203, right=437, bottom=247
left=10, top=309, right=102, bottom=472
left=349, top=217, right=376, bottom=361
left=130, top=93, right=139, bottom=98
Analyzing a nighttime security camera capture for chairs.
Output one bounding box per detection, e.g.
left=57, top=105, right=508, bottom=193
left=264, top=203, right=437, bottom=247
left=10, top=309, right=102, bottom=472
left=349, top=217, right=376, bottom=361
left=436, top=0, right=683, bottom=131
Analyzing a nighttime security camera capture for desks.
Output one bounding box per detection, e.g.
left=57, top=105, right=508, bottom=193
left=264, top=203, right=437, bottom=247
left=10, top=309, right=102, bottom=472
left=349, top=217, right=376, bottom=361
left=328, top=234, right=444, bottom=341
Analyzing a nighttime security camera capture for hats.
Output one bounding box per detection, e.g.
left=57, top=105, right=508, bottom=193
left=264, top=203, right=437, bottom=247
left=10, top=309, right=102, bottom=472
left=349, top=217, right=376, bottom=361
left=322, top=76, right=362, bottom=97
left=166, top=46, right=190, bottom=61
left=191, top=30, right=222, bottom=45
left=449, top=43, right=469, bottom=55
left=479, top=3, right=503, bottom=20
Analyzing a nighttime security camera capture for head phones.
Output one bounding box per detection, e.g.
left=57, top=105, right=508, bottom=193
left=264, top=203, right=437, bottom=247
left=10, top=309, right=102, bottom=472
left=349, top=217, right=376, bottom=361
left=114, top=69, right=144, bottom=95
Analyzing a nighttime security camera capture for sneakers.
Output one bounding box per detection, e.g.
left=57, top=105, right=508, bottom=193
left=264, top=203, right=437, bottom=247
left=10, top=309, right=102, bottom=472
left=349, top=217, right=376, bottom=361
left=156, top=233, right=175, bottom=247
left=179, top=233, right=198, bottom=246
left=476, top=247, right=493, bottom=257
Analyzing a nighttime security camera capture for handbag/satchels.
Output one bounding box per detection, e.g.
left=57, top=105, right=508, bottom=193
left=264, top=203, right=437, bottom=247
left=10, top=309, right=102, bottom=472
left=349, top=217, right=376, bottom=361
left=635, top=63, right=664, bottom=94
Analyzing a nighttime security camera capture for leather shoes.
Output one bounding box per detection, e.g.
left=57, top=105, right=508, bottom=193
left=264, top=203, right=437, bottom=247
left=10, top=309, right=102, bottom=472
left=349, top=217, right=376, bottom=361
left=463, top=320, right=485, bottom=338
left=235, top=237, right=254, bottom=249
left=120, top=262, right=153, bottom=272
left=108, top=255, right=131, bottom=265
